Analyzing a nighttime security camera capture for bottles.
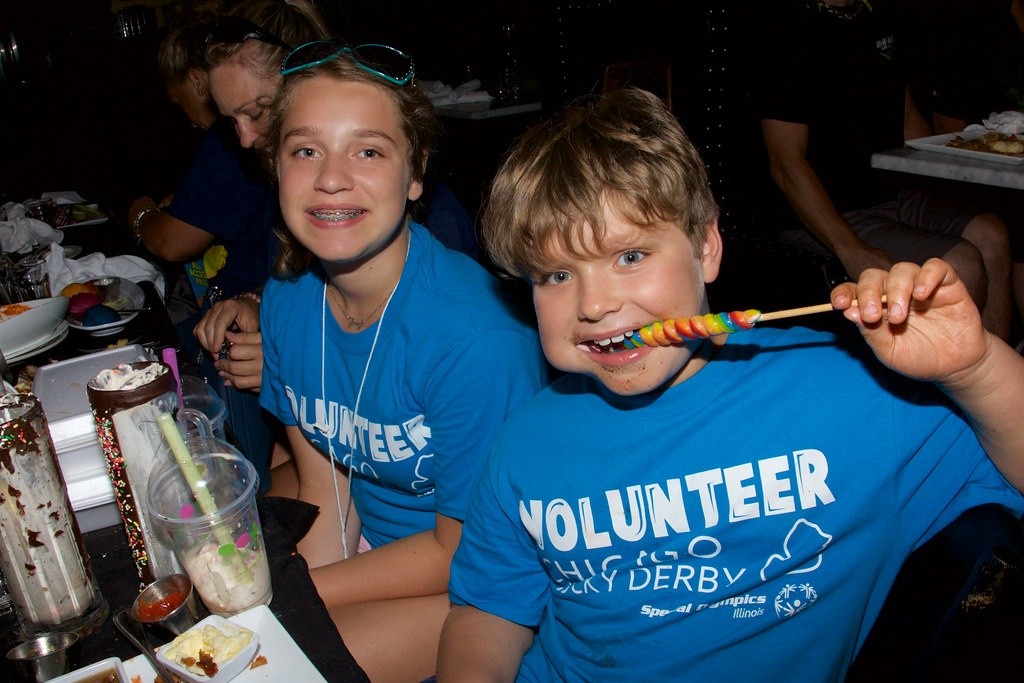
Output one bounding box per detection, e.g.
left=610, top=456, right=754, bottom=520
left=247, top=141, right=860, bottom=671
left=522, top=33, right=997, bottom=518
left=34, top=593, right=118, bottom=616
left=492, top=22, right=521, bottom=106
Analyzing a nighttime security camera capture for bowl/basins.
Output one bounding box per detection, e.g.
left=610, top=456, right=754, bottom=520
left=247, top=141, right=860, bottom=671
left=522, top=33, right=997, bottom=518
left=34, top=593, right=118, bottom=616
left=6, top=631, right=82, bottom=683
left=31, top=344, right=159, bottom=512
left=130, top=573, right=207, bottom=655
left=156, top=614, right=260, bottom=683
left=45, top=656, right=129, bottom=683
left=0, top=297, right=68, bottom=355
left=93, top=276, right=121, bottom=303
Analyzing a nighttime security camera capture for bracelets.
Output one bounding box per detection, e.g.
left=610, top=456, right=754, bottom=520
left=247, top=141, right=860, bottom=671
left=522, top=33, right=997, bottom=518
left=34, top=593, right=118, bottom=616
left=132, top=207, right=161, bottom=239
left=233, top=292, right=261, bottom=309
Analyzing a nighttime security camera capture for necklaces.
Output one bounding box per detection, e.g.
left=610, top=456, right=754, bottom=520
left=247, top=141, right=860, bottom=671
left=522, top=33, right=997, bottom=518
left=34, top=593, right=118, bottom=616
left=817, top=0, right=862, bottom=20
left=322, top=228, right=411, bottom=559
left=328, top=232, right=408, bottom=331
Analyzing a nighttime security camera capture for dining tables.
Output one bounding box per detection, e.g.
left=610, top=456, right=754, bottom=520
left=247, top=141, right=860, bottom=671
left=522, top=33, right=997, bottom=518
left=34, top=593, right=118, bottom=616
left=871, top=145, right=1024, bottom=189
left=0, top=173, right=372, bottom=683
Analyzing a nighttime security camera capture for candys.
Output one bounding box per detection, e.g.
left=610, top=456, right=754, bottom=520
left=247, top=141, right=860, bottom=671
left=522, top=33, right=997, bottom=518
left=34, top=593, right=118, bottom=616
left=587, top=310, right=760, bottom=353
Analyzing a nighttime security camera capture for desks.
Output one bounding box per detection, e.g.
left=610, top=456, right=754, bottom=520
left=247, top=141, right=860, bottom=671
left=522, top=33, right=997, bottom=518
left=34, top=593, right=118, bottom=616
left=431, top=99, right=542, bottom=120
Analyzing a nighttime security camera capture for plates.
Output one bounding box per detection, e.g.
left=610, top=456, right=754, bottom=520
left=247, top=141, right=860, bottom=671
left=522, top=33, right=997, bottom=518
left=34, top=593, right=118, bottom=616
left=4, top=319, right=68, bottom=363
left=905, top=130, right=1024, bottom=164
left=67, top=274, right=145, bottom=338
left=17, top=245, right=82, bottom=266
left=123, top=605, right=327, bottom=683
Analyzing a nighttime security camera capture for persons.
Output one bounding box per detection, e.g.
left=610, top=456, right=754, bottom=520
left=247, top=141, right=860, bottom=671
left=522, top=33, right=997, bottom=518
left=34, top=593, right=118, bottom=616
left=758, top=0, right=1024, bottom=355
left=260, top=39, right=549, bottom=683
left=434, top=86, right=1024, bottom=683
left=128, top=0, right=481, bottom=499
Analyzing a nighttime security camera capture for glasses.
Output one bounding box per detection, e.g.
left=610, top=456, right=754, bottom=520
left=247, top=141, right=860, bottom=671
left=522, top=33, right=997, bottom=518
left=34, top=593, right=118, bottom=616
left=280, top=41, right=415, bottom=88
left=204, top=16, right=295, bottom=52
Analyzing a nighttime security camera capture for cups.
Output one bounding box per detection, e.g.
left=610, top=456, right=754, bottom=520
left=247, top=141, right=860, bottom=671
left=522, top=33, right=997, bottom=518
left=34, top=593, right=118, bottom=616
left=25, top=196, right=56, bottom=230
left=174, top=373, right=230, bottom=443
left=145, top=409, right=272, bottom=617
left=86, top=360, right=219, bottom=585
left=8, top=259, right=52, bottom=302
left=0, top=394, right=109, bottom=640
